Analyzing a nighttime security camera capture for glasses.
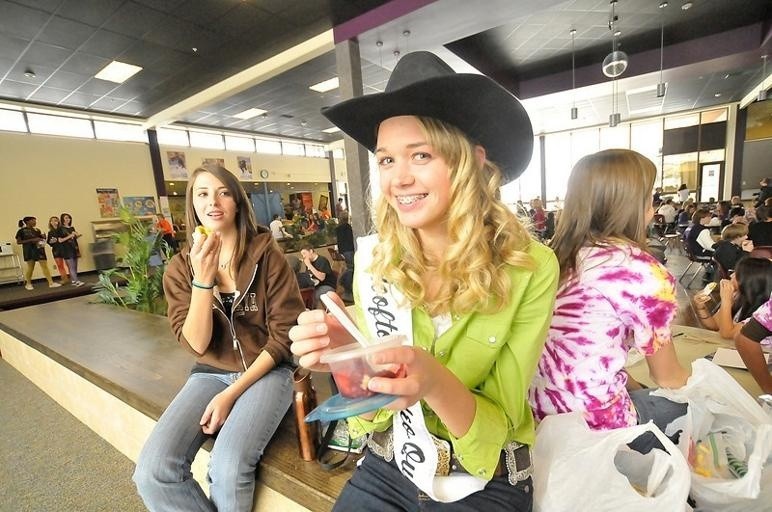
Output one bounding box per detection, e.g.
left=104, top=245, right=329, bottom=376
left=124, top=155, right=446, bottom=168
left=727, top=269, right=736, bottom=276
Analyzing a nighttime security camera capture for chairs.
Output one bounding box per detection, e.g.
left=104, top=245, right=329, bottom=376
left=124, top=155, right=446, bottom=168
left=541, top=211, right=554, bottom=239
left=300, top=287, right=313, bottom=309
left=651, top=206, right=738, bottom=328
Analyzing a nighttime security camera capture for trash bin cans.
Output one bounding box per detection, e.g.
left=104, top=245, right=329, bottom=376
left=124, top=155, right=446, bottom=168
left=88, top=239, right=115, bottom=271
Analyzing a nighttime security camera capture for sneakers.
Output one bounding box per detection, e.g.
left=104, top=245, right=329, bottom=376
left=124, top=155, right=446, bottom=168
left=72, top=281, right=85, bottom=288
left=24, top=285, right=34, bottom=291
left=49, top=283, right=62, bottom=288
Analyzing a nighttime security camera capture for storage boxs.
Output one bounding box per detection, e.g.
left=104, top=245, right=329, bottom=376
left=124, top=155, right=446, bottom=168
left=93, top=250, right=114, bottom=270
left=88, top=240, right=113, bottom=254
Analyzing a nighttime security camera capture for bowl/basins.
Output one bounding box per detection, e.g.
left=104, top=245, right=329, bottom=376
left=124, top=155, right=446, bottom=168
left=318, top=340, right=405, bottom=395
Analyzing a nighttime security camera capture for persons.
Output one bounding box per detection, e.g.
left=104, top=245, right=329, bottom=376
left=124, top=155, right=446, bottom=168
left=15, top=217, right=63, bottom=291
left=652, top=176, right=772, bottom=396
left=47, top=216, right=72, bottom=285
left=287, top=51, right=560, bottom=512
left=524, top=148, right=693, bottom=431
left=153, top=213, right=173, bottom=254
left=513, top=196, right=548, bottom=240
left=57, top=214, right=85, bottom=287
left=130, top=163, right=306, bottom=512
left=268, top=196, right=354, bottom=311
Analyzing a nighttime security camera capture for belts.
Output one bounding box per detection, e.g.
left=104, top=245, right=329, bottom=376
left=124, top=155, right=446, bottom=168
left=366, top=424, right=535, bottom=487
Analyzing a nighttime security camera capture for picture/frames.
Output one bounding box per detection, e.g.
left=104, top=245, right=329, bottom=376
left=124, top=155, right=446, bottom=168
left=317, top=195, right=328, bottom=210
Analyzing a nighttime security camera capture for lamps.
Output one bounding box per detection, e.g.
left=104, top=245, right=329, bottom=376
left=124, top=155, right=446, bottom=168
left=758, top=56, right=770, bottom=100
left=609, top=16, right=618, bottom=127
left=602, top=0, right=629, bottom=78
left=615, top=31, right=621, bottom=125
left=657, top=2, right=668, bottom=97
left=570, top=29, right=577, bottom=119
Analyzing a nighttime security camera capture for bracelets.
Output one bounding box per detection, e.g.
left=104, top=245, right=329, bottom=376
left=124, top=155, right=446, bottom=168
left=192, top=279, right=216, bottom=289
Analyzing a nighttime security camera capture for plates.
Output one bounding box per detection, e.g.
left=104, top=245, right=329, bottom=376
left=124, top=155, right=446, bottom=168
left=304, top=394, right=400, bottom=421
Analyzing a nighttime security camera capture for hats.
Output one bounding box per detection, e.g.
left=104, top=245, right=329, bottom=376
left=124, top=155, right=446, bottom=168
left=319, top=50, right=534, bottom=187
left=286, top=255, right=301, bottom=271
